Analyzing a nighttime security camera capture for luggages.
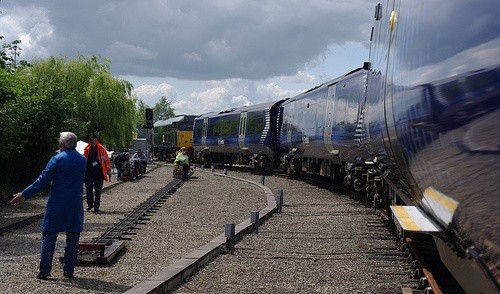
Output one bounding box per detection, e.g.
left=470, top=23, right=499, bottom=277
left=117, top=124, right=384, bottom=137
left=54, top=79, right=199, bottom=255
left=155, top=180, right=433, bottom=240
left=174, top=165, right=184, bottom=179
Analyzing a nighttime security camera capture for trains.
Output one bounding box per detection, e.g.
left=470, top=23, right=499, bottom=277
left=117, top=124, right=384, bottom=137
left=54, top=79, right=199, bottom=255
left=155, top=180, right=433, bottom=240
left=124, top=0, right=500, bottom=294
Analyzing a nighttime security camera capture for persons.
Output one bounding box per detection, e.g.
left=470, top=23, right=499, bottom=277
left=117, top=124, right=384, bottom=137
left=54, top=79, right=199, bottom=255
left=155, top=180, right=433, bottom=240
left=132, top=148, right=147, bottom=172
left=10, top=132, right=86, bottom=280
left=84, top=135, right=112, bottom=211
left=174, top=147, right=189, bottom=167
left=114, top=152, right=129, bottom=181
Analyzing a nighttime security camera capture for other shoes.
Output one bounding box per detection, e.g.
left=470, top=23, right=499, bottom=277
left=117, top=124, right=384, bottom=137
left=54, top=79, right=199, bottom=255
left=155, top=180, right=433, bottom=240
left=116, top=179, right=123, bottom=183
left=37, top=271, right=51, bottom=278
left=86, top=205, right=94, bottom=210
left=94, top=207, right=99, bottom=211
left=63, top=271, right=73, bottom=278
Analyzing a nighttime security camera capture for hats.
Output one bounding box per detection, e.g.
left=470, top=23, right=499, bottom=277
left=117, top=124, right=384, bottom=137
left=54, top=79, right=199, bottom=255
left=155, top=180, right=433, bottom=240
left=179, top=146, right=189, bottom=152
left=88, top=133, right=98, bottom=139
left=135, top=148, right=141, bottom=152
left=126, top=152, right=132, bottom=158
left=129, top=149, right=133, bottom=151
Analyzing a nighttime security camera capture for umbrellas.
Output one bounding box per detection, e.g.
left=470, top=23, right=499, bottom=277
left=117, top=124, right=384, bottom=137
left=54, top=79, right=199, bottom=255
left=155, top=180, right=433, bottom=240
left=55, top=140, right=114, bottom=162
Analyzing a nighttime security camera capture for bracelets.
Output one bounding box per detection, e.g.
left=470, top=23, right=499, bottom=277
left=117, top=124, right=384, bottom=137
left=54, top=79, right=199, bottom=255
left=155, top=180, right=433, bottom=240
left=107, top=169, right=111, bottom=172
